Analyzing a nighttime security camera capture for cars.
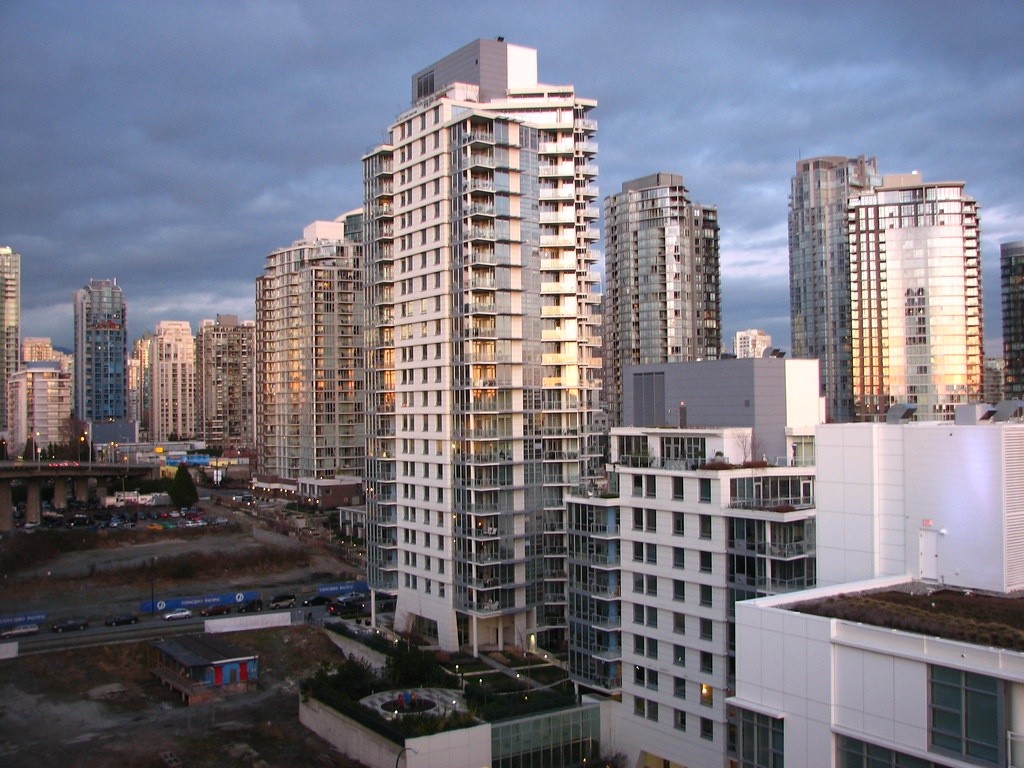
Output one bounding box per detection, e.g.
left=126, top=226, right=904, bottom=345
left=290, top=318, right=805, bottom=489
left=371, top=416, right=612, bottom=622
left=104, top=615, right=139, bottom=627
left=238, top=599, right=264, bottom=614
left=242, top=495, right=256, bottom=507
left=17, top=502, right=228, bottom=532
left=268, top=593, right=297, bottom=610
left=162, top=607, right=194, bottom=621
left=201, top=605, right=231, bottom=617
left=302, top=595, right=331, bottom=608
left=336, top=592, right=366, bottom=603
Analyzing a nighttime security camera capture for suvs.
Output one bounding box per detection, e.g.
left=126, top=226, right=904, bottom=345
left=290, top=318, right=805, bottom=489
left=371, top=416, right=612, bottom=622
left=52, top=617, right=89, bottom=634
left=0, top=624, right=39, bottom=640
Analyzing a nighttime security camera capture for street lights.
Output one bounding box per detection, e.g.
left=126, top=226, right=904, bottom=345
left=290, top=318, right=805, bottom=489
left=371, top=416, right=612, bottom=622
left=78, top=437, right=85, bottom=464
left=32, top=432, right=40, bottom=461
